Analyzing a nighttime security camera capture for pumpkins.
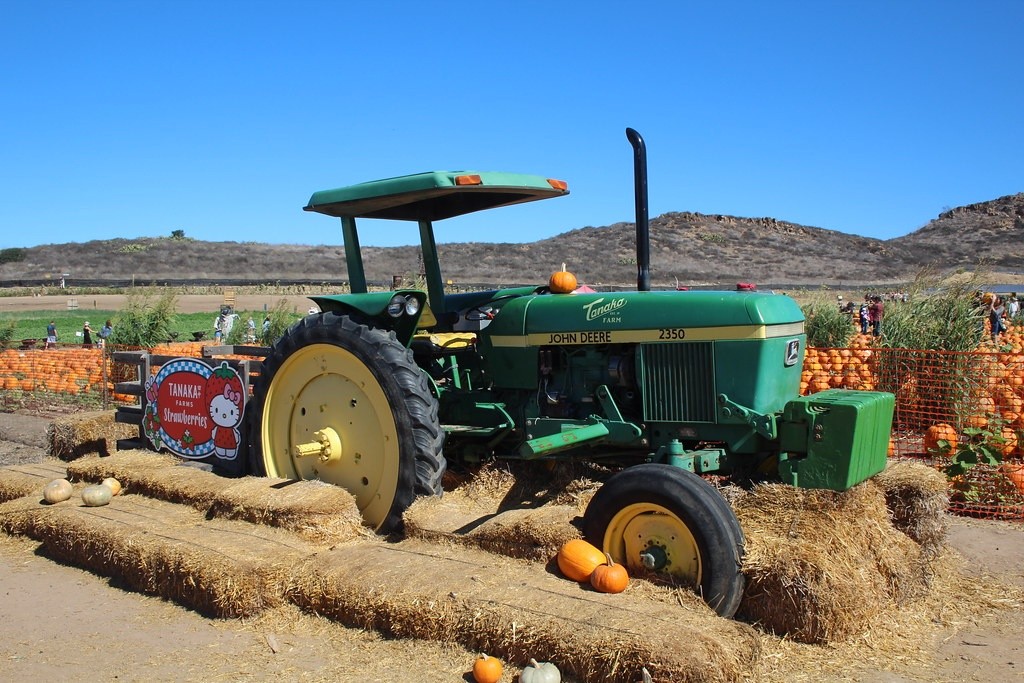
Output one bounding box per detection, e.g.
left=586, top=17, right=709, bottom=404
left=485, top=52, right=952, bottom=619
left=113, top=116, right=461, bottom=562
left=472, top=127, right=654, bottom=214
left=473, top=652, right=502, bottom=683
left=984, top=291, right=995, bottom=304
left=102, top=477, right=121, bottom=496
left=82, top=485, right=112, bottom=508
left=549, top=262, right=577, bottom=293
left=0, top=341, right=267, bottom=407
left=519, top=658, right=561, bottom=683
left=558, top=539, right=607, bottom=584
left=44, top=479, right=73, bottom=504
left=590, top=553, right=628, bottom=593
left=798, top=310, right=1024, bottom=496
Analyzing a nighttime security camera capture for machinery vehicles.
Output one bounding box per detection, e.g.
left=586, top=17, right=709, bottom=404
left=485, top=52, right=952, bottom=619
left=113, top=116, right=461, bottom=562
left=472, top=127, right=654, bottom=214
left=108, top=125, right=898, bottom=625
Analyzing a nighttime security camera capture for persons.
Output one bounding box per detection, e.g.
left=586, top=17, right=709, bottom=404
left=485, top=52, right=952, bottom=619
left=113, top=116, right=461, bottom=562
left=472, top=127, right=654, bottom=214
left=82, top=321, right=95, bottom=348
left=213, top=306, right=272, bottom=343
left=838, top=286, right=1019, bottom=346
left=45, top=320, right=58, bottom=349
left=100, top=319, right=114, bottom=338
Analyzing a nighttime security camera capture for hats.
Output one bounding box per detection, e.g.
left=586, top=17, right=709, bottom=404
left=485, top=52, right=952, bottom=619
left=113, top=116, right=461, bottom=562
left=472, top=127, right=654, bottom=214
left=85, top=321, right=90, bottom=326
